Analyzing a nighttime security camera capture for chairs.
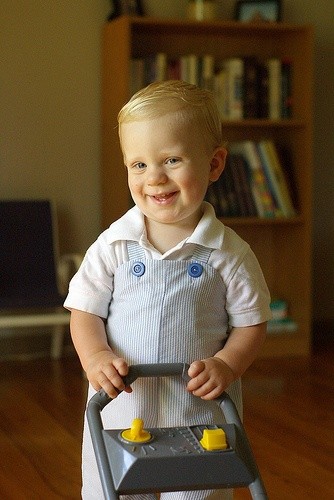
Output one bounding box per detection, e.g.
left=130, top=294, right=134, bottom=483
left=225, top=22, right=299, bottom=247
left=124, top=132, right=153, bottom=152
left=0, top=199, right=88, bottom=378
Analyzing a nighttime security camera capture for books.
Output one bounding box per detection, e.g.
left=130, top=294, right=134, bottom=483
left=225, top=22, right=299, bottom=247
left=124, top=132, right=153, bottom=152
left=202, top=138, right=296, bottom=219
left=129, top=51, right=298, bottom=123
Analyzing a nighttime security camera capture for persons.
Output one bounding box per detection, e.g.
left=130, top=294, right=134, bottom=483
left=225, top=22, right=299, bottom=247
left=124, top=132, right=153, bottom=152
left=62, top=80, right=273, bottom=500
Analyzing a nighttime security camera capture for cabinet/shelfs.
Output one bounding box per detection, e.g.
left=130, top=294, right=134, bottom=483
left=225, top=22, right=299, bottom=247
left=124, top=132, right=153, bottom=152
left=103, top=16, right=313, bottom=357
